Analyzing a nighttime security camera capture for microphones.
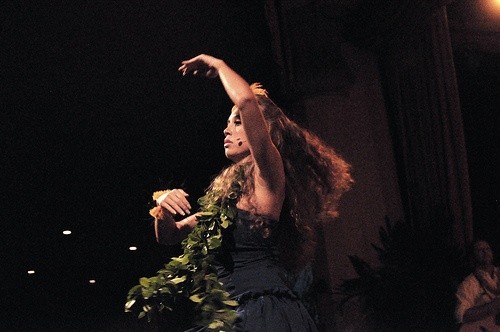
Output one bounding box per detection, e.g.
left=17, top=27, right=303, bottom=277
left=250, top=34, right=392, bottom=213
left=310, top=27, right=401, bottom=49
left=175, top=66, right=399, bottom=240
left=237, top=136, right=241, bottom=141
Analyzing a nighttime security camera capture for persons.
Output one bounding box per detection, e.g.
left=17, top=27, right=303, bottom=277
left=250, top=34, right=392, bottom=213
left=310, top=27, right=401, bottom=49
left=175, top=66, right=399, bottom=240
left=454, top=241, right=500, bottom=332
left=148, top=54, right=353, bottom=332
